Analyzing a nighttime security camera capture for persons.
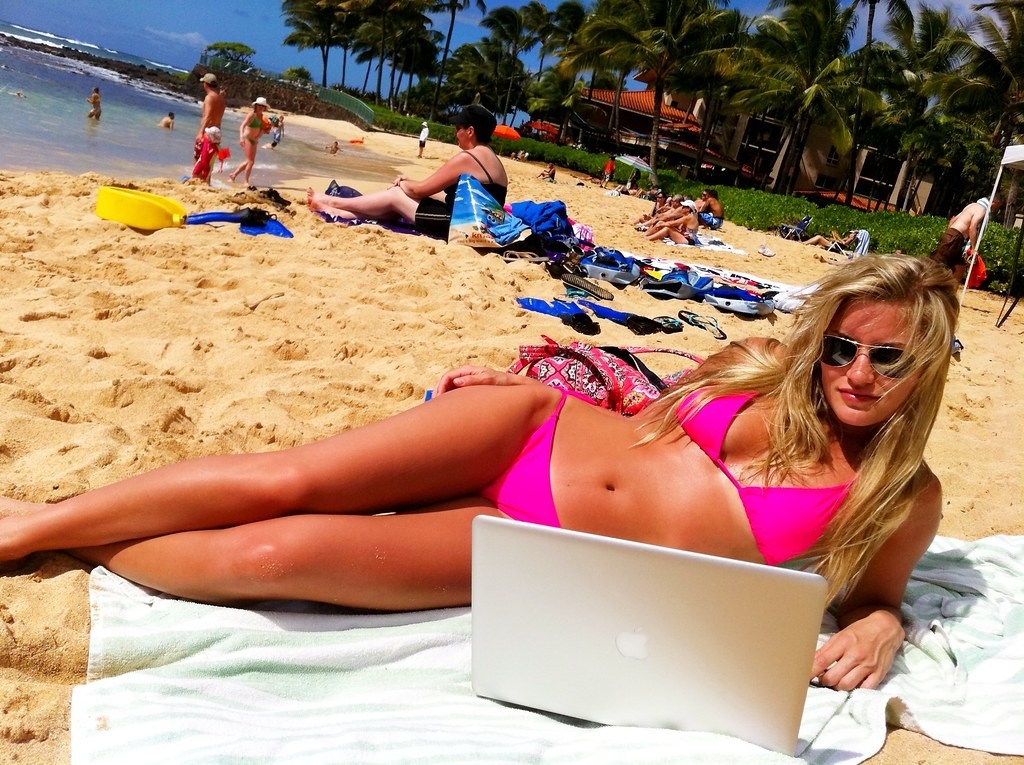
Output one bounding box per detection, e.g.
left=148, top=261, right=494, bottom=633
left=161, top=112, right=174, bottom=129
left=229, top=97, right=271, bottom=185
left=627, top=168, right=724, bottom=246
left=87, top=87, right=101, bottom=120
left=307, top=105, right=508, bottom=240
left=417, top=122, right=429, bottom=158
left=599, top=157, right=616, bottom=189
left=264, top=141, right=277, bottom=149
left=511, top=149, right=556, bottom=182
left=615, top=184, right=639, bottom=196
left=325, top=141, right=341, bottom=154
left=274, top=115, right=284, bottom=143
left=1, top=254, right=959, bottom=693
left=803, top=232, right=858, bottom=254
left=193, top=74, right=226, bottom=186
left=930, top=194, right=1003, bottom=281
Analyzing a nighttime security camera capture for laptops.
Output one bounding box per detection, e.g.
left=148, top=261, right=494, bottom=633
left=469, top=515, right=850, bottom=756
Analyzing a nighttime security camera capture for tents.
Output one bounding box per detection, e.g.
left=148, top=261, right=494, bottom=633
left=960, top=144, right=1024, bottom=308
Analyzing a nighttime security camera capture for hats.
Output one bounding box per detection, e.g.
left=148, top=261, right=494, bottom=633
left=200, top=73, right=218, bottom=85
left=252, top=97, right=270, bottom=109
left=205, top=126, right=222, bottom=143
left=421, top=121, right=428, bottom=128
left=680, top=200, right=696, bottom=212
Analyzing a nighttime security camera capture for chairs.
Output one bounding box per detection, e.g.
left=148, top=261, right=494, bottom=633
left=826, top=230, right=865, bottom=258
left=513, top=152, right=529, bottom=161
left=782, top=216, right=814, bottom=242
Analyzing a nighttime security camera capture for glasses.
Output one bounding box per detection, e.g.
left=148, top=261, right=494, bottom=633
left=817, top=335, right=917, bottom=380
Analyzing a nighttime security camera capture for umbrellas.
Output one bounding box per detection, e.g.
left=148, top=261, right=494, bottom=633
left=616, top=156, right=655, bottom=183
left=526, top=120, right=558, bottom=135
left=493, top=125, right=520, bottom=156
